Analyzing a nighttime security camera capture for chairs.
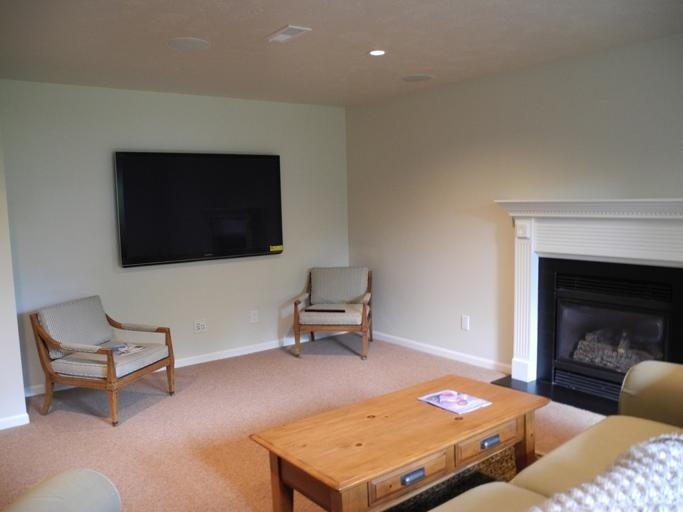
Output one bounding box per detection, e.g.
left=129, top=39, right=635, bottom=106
left=29, top=295, right=174, bottom=426
left=0, top=467, right=121, bottom=512
left=295, top=267, right=372, bottom=359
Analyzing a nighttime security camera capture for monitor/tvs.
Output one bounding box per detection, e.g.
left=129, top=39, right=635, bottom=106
left=112, top=149, right=283, bottom=268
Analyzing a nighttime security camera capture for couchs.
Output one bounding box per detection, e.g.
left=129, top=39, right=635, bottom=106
left=424, top=361, right=683, bottom=512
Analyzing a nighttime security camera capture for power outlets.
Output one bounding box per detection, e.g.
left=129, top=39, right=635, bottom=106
left=462, top=316, right=470, bottom=331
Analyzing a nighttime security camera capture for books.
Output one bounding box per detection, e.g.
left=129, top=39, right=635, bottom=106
left=110, top=342, right=144, bottom=357
left=416, top=389, right=492, bottom=414
